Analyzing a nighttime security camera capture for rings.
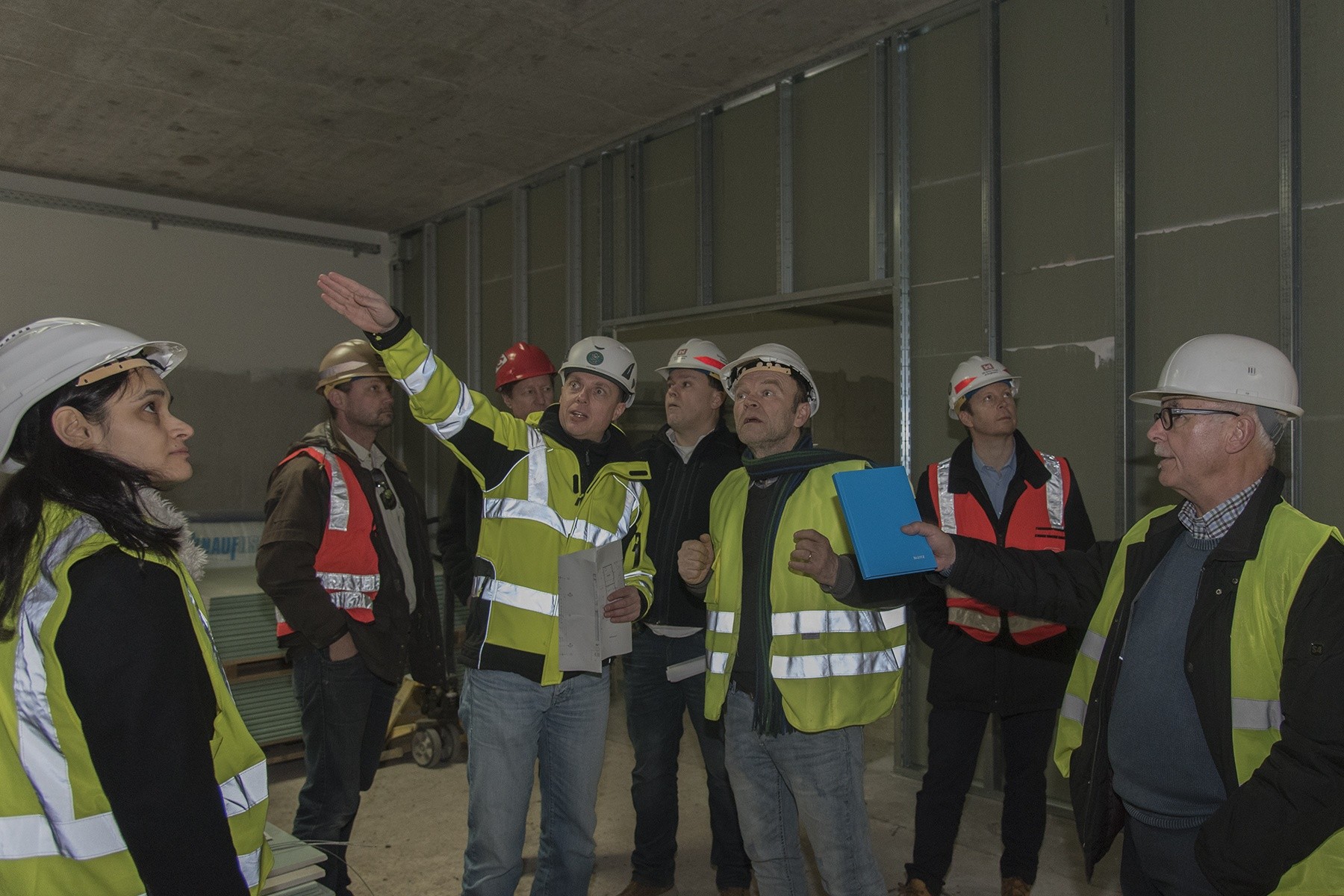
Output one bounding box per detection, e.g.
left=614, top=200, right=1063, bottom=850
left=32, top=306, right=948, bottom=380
left=805, top=551, right=812, bottom=561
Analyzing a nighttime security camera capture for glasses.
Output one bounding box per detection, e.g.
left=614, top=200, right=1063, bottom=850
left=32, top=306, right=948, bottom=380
left=370, top=467, right=396, bottom=510
left=1154, top=407, right=1239, bottom=431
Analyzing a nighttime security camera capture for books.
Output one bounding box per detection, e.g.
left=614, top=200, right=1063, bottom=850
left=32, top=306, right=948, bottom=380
left=832, top=466, right=939, bottom=580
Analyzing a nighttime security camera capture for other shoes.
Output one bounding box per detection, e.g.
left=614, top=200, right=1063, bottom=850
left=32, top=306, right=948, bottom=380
left=617, top=870, right=676, bottom=896
left=896, top=879, right=932, bottom=896
left=1001, top=875, right=1031, bottom=896
left=715, top=864, right=754, bottom=896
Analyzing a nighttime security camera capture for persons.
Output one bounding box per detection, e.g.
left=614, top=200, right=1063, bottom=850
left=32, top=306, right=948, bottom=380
left=678, top=343, right=932, bottom=896
left=1054, top=333, right=1344, bottom=896
left=0, top=317, right=277, bottom=896
left=889, top=355, right=1096, bottom=896
left=256, top=339, right=448, bottom=895
left=319, top=272, right=657, bottom=896
left=435, top=341, right=558, bottom=881
left=621, top=339, right=748, bottom=896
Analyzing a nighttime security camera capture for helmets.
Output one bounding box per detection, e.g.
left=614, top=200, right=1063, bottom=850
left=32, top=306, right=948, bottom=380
left=653, top=338, right=732, bottom=395
left=948, top=355, right=1021, bottom=413
left=495, top=341, right=558, bottom=391
left=0, top=317, right=187, bottom=474
left=719, top=343, right=819, bottom=420
left=1128, top=334, right=1304, bottom=419
left=315, top=339, right=389, bottom=393
left=557, top=336, right=638, bottom=410
